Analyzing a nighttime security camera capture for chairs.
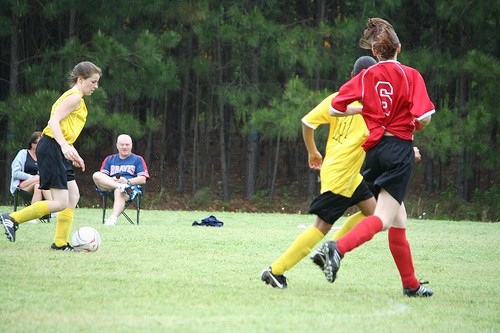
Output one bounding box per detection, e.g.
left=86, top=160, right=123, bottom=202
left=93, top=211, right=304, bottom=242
left=14, top=185, right=50, bottom=223
left=95, top=186, right=142, bottom=225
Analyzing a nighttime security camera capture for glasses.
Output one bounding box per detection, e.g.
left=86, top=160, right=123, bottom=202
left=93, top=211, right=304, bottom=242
left=31, top=141, right=38, bottom=144
left=116, top=142, right=132, bottom=147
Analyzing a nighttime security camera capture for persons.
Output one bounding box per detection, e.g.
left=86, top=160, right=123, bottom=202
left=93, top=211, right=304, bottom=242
left=10, top=132, right=56, bottom=224
left=0, top=61, right=102, bottom=252
left=93, top=134, right=149, bottom=225
left=261, top=56, right=421, bottom=289
left=321, top=18, right=433, bottom=298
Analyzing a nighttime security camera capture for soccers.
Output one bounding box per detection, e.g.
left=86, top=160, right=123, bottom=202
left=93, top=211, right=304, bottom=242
left=72, top=227, right=100, bottom=252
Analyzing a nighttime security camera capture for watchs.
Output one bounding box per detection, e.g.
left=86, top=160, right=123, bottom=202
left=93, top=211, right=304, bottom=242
left=127, top=179, right=131, bottom=185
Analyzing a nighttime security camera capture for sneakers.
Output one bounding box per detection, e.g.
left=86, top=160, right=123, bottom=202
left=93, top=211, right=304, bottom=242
left=311, top=248, right=326, bottom=271
left=0, top=212, right=19, bottom=242
left=105, top=214, right=118, bottom=225
left=261, top=267, right=289, bottom=290
left=126, top=183, right=143, bottom=201
left=322, top=240, right=344, bottom=284
left=402, top=280, right=434, bottom=298
left=51, top=242, right=75, bottom=252
